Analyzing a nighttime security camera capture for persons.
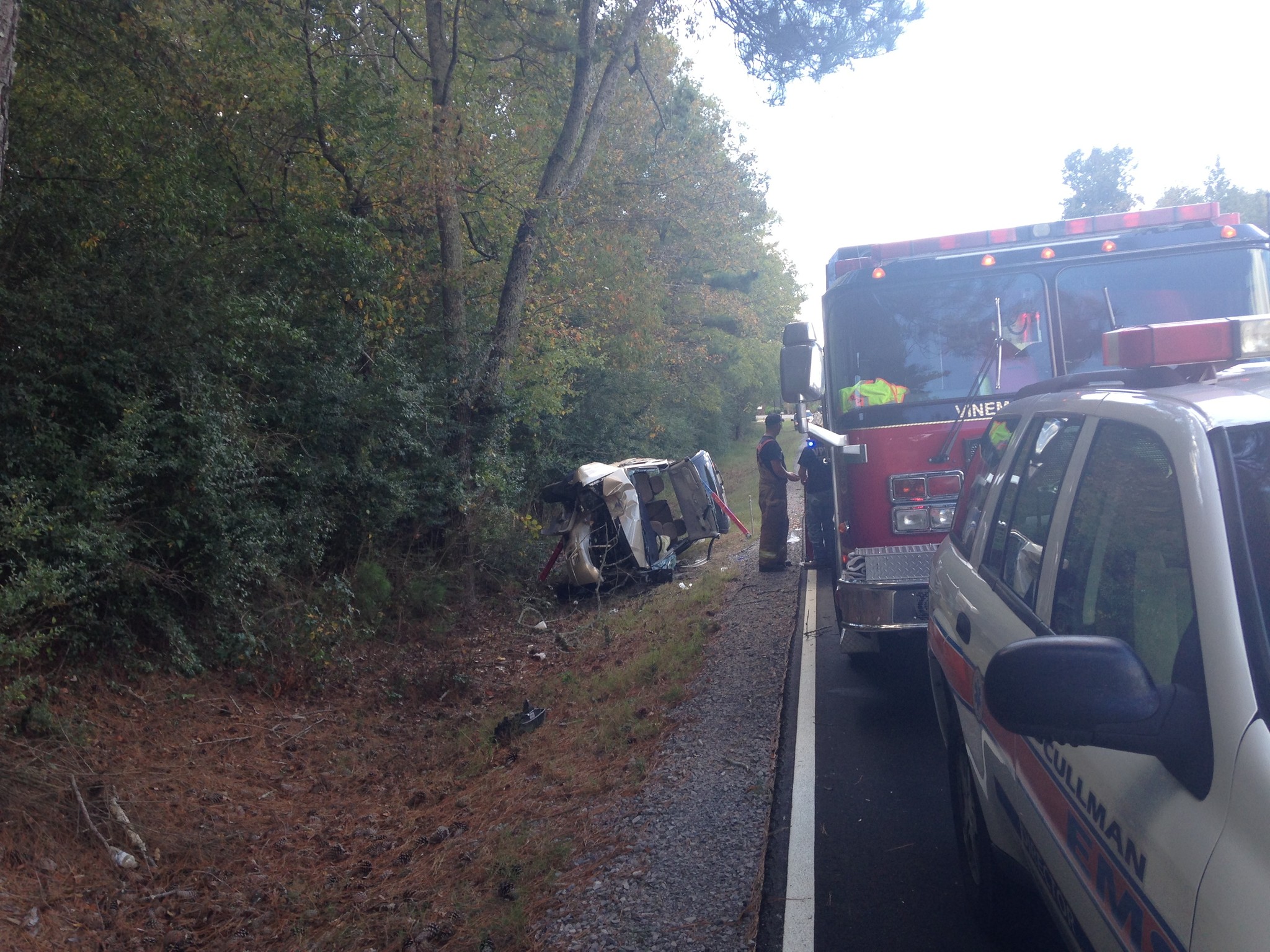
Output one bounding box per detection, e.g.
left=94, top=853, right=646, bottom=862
left=797, top=424, right=838, bottom=570
left=756, top=414, right=801, bottom=572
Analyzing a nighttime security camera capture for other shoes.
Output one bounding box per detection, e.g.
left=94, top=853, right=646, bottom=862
left=785, top=560, right=792, bottom=566
left=825, top=560, right=835, bottom=568
left=759, top=565, right=788, bottom=572
left=804, top=558, right=825, bottom=569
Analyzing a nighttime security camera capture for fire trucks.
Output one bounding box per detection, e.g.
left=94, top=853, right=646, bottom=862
left=777, top=204, right=1270, bottom=664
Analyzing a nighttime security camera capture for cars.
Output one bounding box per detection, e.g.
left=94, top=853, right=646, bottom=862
left=929, top=313, right=1269, bottom=951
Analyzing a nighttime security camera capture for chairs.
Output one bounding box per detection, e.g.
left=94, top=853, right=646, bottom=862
left=650, top=521, right=678, bottom=544
left=632, top=473, right=665, bottom=505
left=646, top=500, right=688, bottom=537
